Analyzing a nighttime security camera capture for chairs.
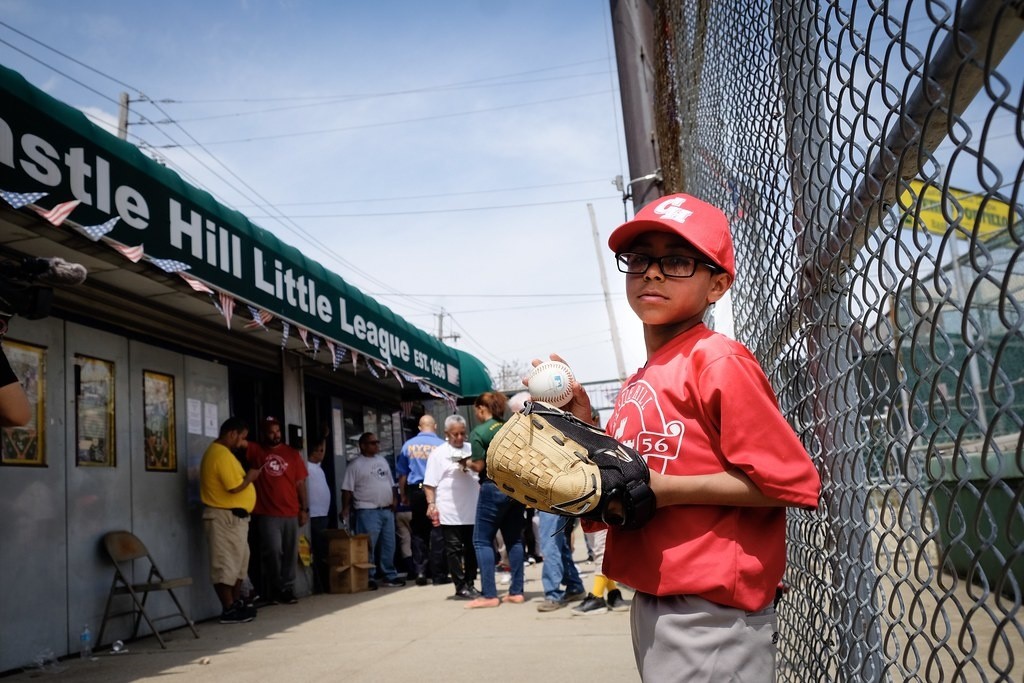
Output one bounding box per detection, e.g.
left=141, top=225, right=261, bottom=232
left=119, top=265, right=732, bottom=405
left=93, top=530, right=199, bottom=648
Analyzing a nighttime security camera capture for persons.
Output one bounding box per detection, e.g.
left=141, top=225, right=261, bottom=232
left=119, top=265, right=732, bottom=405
left=199, top=416, right=331, bottom=623
left=340, top=432, right=407, bottom=591
left=395, top=391, right=629, bottom=615
left=0, top=343, right=33, bottom=427
left=523, top=194, right=821, bottom=683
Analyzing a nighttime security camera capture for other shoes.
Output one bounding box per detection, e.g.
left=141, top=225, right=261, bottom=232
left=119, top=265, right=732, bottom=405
left=439, top=577, right=452, bottom=584
left=278, top=587, right=298, bottom=604
left=415, top=574, right=427, bottom=584
left=455, top=584, right=482, bottom=600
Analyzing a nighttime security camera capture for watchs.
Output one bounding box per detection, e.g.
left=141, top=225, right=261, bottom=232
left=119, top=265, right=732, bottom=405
left=300, top=506, right=310, bottom=513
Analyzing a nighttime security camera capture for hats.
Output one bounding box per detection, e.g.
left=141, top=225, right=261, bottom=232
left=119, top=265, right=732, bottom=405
left=608, top=193, right=736, bottom=284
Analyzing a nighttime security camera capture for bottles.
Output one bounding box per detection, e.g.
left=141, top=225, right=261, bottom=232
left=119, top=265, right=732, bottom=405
left=80, top=624, right=92, bottom=661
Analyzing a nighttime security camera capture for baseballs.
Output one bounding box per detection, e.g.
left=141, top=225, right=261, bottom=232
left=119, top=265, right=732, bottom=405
left=529, top=358, right=578, bottom=409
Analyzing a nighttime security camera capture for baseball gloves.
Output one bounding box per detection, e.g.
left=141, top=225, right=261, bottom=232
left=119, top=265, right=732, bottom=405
left=481, top=402, right=657, bottom=533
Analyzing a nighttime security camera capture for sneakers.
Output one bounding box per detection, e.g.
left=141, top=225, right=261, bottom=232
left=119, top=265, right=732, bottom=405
left=368, top=575, right=378, bottom=590
left=502, top=594, right=525, bottom=603
left=563, top=588, right=586, bottom=602
left=472, top=596, right=500, bottom=608
left=219, top=600, right=253, bottom=623
left=606, top=589, right=629, bottom=611
left=538, top=589, right=568, bottom=612
left=382, top=578, right=406, bottom=587
left=574, top=591, right=606, bottom=611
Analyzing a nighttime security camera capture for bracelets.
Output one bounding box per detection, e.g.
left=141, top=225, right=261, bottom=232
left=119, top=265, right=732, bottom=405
left=427, top=502, right=436, bottom=505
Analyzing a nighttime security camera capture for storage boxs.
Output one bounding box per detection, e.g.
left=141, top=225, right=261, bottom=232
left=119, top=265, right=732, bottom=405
left=323, top=528, right=377, bottom=594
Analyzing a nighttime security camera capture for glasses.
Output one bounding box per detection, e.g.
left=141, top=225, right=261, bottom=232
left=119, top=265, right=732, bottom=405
left=367, top=440, right=380, bottom=445
left=615, top=250, right=725, bottom=278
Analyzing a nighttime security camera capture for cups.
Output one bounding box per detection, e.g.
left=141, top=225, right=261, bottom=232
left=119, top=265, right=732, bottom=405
left=430, top=511, right=439, bottom=527
